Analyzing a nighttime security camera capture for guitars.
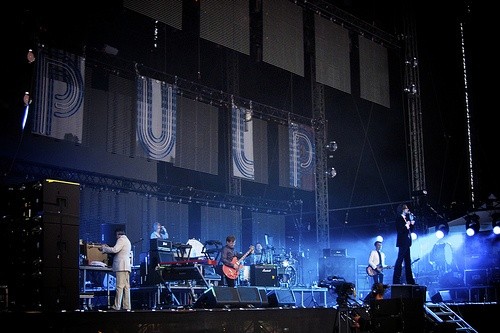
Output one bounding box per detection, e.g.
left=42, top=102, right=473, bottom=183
left=402, top=257, right=420, bottom=276
left=366, top=264, right=394, bottom=277
left=223, top=245, right=255, bottom=279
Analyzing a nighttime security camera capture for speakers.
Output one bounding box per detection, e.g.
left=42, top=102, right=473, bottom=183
left=318, top=257, right=357, bottom=291
left=17, top=181, right=81, bottom=314
left=150, top=251, right=176, bottom=265
left=193, top=285, right=297, bottom=309
left=80, top=244, right=109, bottom=266
left=250, top=264, right=279, bottom=287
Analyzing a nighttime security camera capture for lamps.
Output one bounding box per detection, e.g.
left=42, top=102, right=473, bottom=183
left=24, top=91, right=32, bottom=106
left=331, top=167, right=337, bottom=177
left=464, top=214, right=481, bottom=236
left=491, top=212, right=500, bottom=234
left=435, top=220, right=449, bottom=238
left=28, top=49, right=35, bottom=62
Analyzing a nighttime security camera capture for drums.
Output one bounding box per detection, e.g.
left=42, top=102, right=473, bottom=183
left=291, top=251, right=304, bottom=258
left=272, top=254, right=282, bottom=268
left=277, top=265, right=297, bottom=286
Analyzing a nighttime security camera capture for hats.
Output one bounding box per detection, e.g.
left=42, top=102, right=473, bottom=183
left=226, top=236, right=236, bottom=241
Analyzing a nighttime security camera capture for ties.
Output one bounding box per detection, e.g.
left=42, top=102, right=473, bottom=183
left=378, top=251, right=382, bottom=266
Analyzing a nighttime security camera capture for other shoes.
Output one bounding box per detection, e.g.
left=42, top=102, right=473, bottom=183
left=105, top=308, right=118, bottom=312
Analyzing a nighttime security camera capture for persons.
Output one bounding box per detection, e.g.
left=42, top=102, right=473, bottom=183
left=183, top=233, right=208, bottom=257
left=353, top=283, right=387, bottom=333
left=98, top=228, right=131, bottom=311
left=392, top=203, right=418, bottom=285
left=150, top=222, right=169, bottom=239
left=221, top=235, right=254, bottom=288
left=368, top=241, right=389, bottom=299
left=255, top=243, right=262, bottom=255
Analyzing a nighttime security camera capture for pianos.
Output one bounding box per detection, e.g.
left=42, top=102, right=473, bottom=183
left=172, top=245, right=192, bottom=265
